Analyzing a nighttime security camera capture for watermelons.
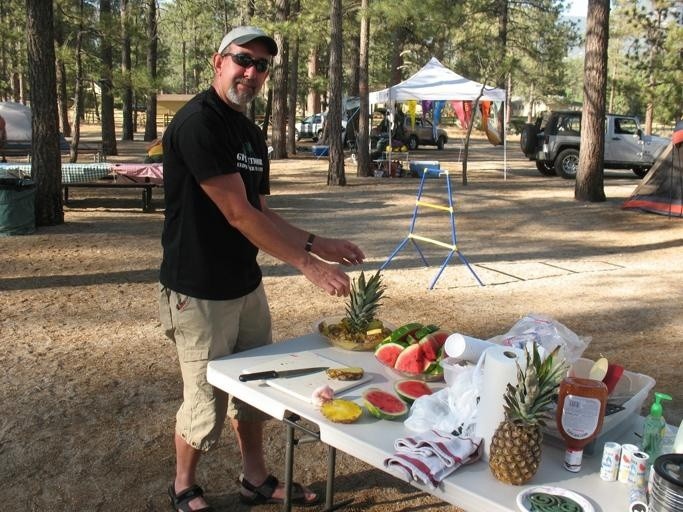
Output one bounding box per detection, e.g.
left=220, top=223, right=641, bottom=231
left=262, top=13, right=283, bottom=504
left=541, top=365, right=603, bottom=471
left=363, top=387, right=409, bottom=420
left=375, top=323, right=452, bottom=380
left=395, top=379, right=432, bottom=404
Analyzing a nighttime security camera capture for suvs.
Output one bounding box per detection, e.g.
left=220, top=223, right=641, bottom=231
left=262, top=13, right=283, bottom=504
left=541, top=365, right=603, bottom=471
left=520, top=108, right=670, bottom=181
left=294, top=113, right=347, bottom=141
left=372, top=108, right=446, bottom=151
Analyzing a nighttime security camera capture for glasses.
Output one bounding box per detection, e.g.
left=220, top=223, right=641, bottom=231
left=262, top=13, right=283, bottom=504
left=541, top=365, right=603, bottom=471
left=223, top=52, right=269, bottom=74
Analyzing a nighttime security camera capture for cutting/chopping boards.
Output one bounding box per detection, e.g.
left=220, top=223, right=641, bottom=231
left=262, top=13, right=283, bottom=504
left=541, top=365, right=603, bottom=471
left=240, top=350, right=374, bottom=405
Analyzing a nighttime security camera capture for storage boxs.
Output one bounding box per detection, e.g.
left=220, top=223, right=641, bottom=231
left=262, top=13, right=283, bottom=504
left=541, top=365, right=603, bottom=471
left=313, top=146, right=329, bottom=155
left=410, top=161, right=440, bottom=178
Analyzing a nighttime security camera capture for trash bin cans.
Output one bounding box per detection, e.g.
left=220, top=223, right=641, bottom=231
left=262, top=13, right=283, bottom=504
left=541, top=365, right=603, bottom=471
left=0, top=178, right=36, bottom=236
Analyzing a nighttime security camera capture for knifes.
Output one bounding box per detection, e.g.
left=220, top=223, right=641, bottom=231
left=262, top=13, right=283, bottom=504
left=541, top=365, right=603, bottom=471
left=238, top=366, right=331, bottom=382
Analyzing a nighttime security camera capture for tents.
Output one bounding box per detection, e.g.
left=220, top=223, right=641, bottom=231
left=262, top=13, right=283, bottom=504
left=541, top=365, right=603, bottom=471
left=617, top=109, right=682, bottom=219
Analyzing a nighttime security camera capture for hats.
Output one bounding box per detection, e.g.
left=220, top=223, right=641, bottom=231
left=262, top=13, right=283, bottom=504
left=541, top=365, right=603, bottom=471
left=217, top=24, right=278, bottom=58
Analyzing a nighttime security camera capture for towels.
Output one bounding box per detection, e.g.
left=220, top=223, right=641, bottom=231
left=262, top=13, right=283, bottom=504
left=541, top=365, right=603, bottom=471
left=383, top=429, right=485, bottom=490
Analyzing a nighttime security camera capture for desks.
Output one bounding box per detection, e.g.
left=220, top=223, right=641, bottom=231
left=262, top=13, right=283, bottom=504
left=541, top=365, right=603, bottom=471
left=207, top=331, right=683, bottom=512
left=382, top=151, right=410, bottom=161
left=372, top=159, right=394, bottom=169
left=0, top=163, right=163, bottom=211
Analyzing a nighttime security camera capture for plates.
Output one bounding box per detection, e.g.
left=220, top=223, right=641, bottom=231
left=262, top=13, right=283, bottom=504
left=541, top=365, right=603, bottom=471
left=373, top=343, right=445, bottom=383
left=514, top=484, right=597, bottom=512
left=310, top=314, right=397, bottom=352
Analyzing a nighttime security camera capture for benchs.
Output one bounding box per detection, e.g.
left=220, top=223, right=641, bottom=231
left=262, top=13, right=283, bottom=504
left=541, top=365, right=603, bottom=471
left=0, top=137, right=107, bottom=163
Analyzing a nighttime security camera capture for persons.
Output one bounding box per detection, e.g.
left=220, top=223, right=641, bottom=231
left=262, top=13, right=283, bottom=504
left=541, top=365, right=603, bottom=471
left=153, top=25, right=365, bottom=512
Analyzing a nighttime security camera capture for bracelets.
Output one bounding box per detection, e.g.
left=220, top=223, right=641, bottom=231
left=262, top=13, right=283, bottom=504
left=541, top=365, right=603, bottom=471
left=304, top=234, right=315, bottom=253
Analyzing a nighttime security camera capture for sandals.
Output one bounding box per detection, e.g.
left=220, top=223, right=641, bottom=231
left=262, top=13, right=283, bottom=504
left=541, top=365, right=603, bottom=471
left=166, top=479, right=217, bottom=512
left=235, top=472, right=323, bottom=508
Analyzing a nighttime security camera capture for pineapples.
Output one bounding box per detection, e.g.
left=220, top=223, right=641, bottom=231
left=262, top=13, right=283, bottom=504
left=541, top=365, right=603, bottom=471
left=325, top=367, right=363, bottom=381
left=321, top=398, right=363, bottom=423
left=319, top=273, right=391, bottom=351
left=488, top=343, right=570, bottom=485
